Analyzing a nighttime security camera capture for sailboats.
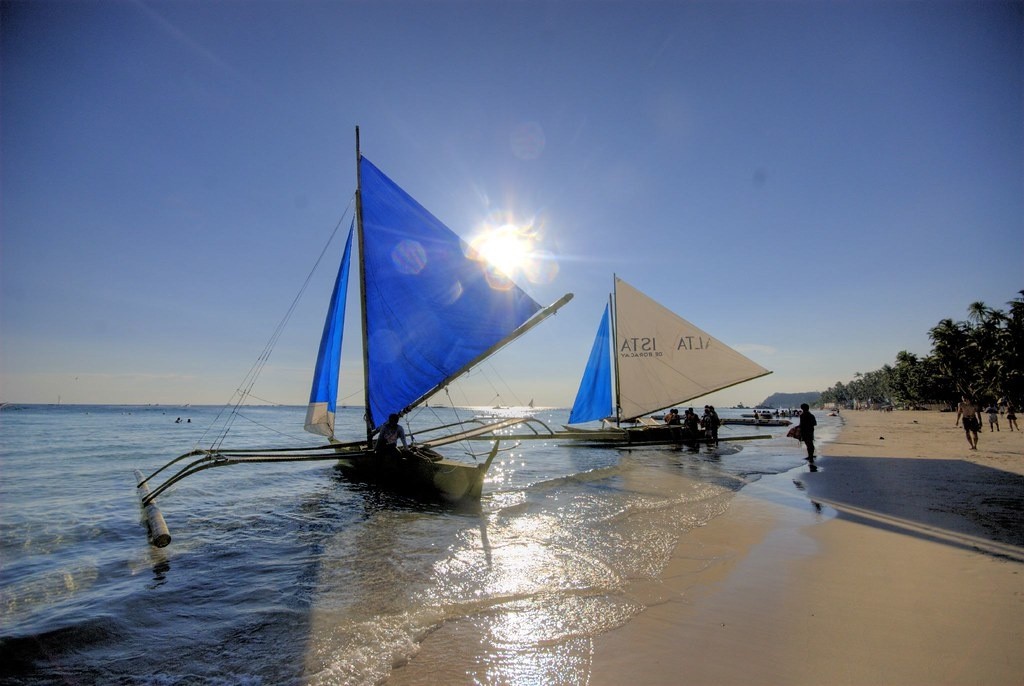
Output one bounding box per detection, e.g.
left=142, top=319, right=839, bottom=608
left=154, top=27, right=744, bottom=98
left=135, top=124, right=629, bottom=546
left=561, top=270, right=774, bottom=441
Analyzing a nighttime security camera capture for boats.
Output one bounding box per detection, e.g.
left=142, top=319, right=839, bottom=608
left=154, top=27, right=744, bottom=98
left=740, top=413, right=800, bottom=417
left=491, top=405, right=508, bottom=409
left=650, top=415, right=793, bottom=426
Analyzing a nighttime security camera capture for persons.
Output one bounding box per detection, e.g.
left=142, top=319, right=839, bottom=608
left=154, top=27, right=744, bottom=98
left=984, top=403, right=1000, bottom=433
left=955, top=394, right=982, bottom=451
left=799, top=404, right=818, bottom=464
left=176, top=417, right=192, bottom=424
left=753, top=408, right=802, bottom=419
left=372, top=413, right=411, bottom=463
left=665, top=405, right=720, bottom=449
left=1004, top=401, right=1020, bottom=432
